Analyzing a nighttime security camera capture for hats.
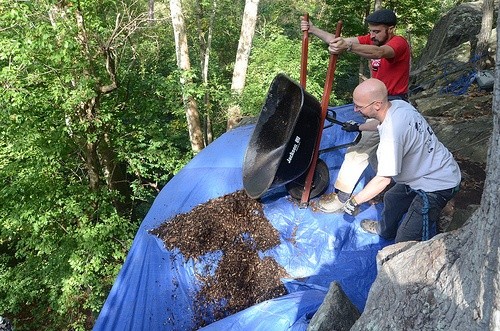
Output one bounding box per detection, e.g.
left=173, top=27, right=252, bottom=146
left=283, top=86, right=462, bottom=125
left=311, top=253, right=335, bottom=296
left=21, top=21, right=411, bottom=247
left=365, top=9, right=396, bottom=27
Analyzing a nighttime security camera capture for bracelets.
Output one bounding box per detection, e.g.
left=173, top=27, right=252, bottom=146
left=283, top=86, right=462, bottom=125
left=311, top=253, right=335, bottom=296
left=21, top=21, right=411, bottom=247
left=346, top=41, right=353, bottom=53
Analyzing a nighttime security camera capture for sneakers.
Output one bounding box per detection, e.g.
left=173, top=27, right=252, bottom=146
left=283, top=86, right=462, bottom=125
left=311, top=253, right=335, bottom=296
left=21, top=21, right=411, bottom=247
left=360, top=219, right=379, bottom=234
left=318, top=194, right=351, bottom=212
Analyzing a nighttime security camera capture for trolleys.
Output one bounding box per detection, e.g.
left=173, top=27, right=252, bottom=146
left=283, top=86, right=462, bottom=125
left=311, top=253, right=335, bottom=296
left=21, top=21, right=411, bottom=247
left=241, top=13, right=363, bottom=210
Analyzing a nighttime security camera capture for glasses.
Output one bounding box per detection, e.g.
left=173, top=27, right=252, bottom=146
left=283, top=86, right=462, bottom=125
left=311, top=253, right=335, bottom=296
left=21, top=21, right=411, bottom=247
left=354, top=101, right=383, bottom=112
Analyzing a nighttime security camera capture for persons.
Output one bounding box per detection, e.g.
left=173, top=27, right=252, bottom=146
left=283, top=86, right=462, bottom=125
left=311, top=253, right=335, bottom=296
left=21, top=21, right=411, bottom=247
left=300, top=8, right=410, bottom=213
left=343, top=78, right=462, bottom=243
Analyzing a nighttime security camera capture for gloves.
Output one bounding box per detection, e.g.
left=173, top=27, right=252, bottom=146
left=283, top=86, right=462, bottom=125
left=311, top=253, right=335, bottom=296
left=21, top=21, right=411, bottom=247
left=344, top=195, right=359, bottom=216
left=341, top=120, right=362, bottom=133
